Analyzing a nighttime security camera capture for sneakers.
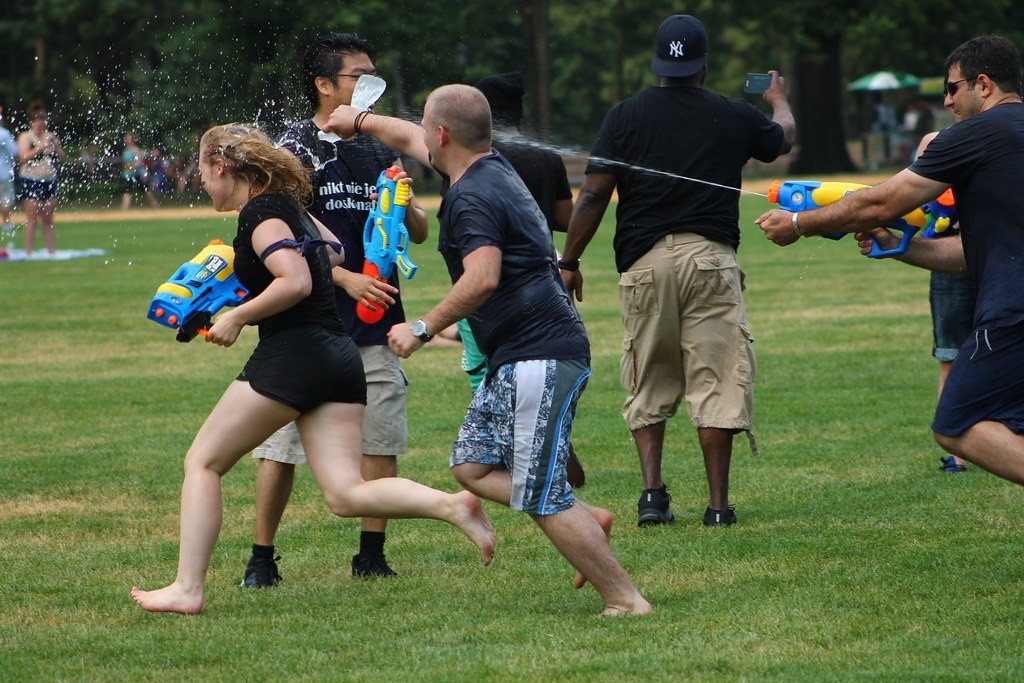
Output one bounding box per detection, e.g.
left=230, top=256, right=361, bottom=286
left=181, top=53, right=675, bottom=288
left=638, top=484, right=674, bottom=525
left=703, top=505, right=736, bottom=526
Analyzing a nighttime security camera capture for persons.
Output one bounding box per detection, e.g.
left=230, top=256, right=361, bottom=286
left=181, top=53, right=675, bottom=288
left=558, top=15, right=796, bottom=528
left=242, top=32, right=428, bottom=588
left=320, top=71, right=651, bottom=616
left=132, top=123, right=496, bottom=614
left=754, top=35, right=1024, bottom=487
left=72, top=133, right=200, bottom=213
left=0, top=101, right=65, bottom=260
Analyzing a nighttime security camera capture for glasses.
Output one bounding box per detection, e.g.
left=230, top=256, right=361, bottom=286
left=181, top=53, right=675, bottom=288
left=944, top=77, right=977, bottom=96
left=334, top=73, right=383, bottom=83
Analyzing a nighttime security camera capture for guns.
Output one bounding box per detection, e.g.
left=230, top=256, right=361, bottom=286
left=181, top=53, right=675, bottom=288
left=144, top=238, right=250, bottom=346
left=354, top=163, right=418, bottom=326
left=767, top=179, right=957, bottom=260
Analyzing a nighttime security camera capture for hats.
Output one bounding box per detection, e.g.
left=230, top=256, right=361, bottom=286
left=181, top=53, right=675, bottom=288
left=474, top=72, right=525, bottom=125
left=650, top=14, right=706, bottom=76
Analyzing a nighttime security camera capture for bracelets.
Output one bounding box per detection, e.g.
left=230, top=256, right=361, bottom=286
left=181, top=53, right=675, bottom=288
left=792, top=213, right=801, bottom=238
left=354, top=110, right=374, bottom=135
left=558, top=257, right=581, bottom=271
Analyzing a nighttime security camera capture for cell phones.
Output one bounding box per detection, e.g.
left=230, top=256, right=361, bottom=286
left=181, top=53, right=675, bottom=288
left=744, top=73, right=772, bottom=93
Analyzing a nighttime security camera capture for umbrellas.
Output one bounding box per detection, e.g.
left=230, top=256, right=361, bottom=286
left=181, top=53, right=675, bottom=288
left=847, top=69, right=919, bottom=90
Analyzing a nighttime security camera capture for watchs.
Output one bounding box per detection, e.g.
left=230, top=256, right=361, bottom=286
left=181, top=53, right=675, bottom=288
left=411, top=318, right=434, bottom=342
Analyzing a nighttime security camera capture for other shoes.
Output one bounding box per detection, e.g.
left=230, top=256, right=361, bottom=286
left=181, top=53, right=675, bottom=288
left=353, top=553, right=397, bottom=579
left=942, top=455, right=967, bottom=472
left=241, top=554, right=281, bottom=587
